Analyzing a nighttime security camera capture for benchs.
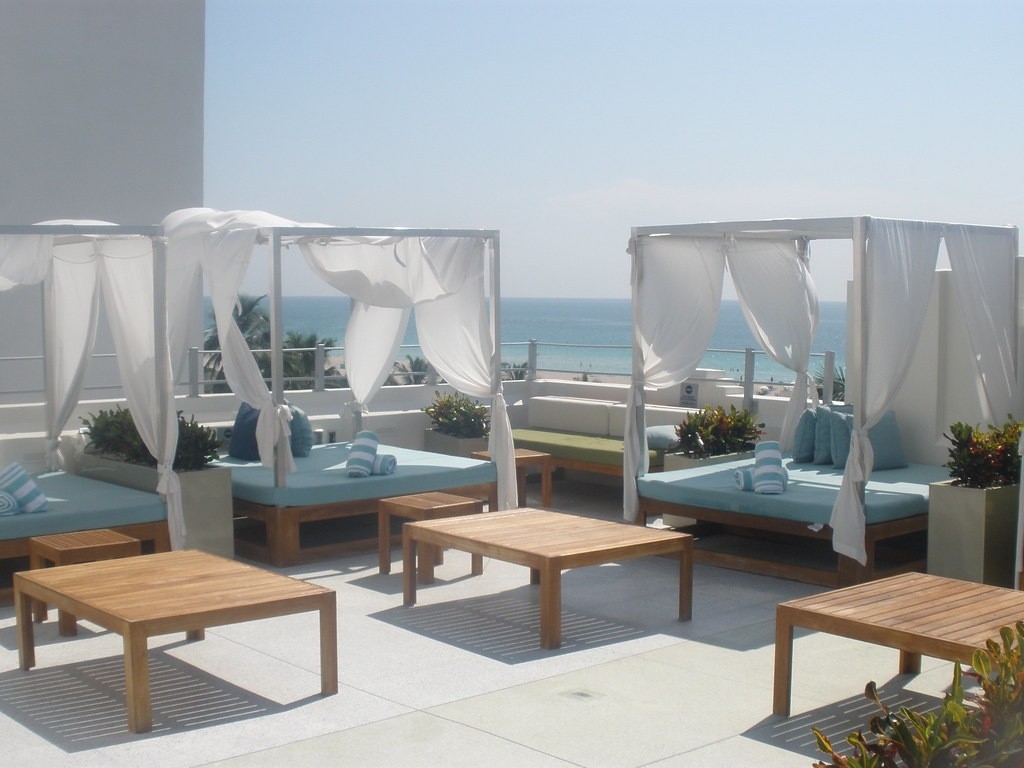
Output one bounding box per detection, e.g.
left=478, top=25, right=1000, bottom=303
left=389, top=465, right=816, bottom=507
left=512, top=395, right=719, bottom=476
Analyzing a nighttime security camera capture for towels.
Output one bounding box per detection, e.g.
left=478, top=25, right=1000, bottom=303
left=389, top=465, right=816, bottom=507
left=0, top=461, right=49, bottom=513
left=345, top=430, right=380, bottom=478
left=0, top=490, right=20, bottom=517
left=755, top=440, right=783, bottom=495
left=734, top=466, right=789, bottom=491
left=372, top=454, right=397, bottom=475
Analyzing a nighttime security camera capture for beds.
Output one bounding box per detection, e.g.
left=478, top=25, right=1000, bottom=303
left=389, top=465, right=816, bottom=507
left=0, top=208, right=520, bottom=607
left=622, top=216, right=1024, bottom=590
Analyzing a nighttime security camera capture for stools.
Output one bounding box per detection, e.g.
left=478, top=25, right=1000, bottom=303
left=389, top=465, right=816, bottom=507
left=29, top=529, right=141, bottom=624
left=378, top=492, right=483, bottom=583
left=470, top=448, right=552, bottom=509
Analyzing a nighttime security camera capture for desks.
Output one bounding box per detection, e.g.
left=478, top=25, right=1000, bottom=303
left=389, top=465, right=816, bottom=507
left=14, top=549, right=338, bottom=735
left=774, top=571, right=1024, bottom=718
left=403, top=508, right=694, bottom=649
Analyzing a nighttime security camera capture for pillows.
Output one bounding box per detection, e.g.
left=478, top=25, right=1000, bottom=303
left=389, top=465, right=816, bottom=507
left=832, top=411, right=907, bottom=468
left=814, top=405, right=853, bottom=464
left=645, top=425, right=682, bottom=449
left=229, top=402, right=261, bottom=462
left=283, top=398, right=313, bottom=457
left=792, top=408, right=817, bottom=462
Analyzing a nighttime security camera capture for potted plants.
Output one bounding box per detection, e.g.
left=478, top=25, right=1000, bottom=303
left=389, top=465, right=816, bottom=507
left=663, top=400, right=767, bottom=528
left=421, top=390, right=491, bottom=458
left=927, top=414, right=1023, bottom=591
left=79, top=403, right=235, bottom=562
